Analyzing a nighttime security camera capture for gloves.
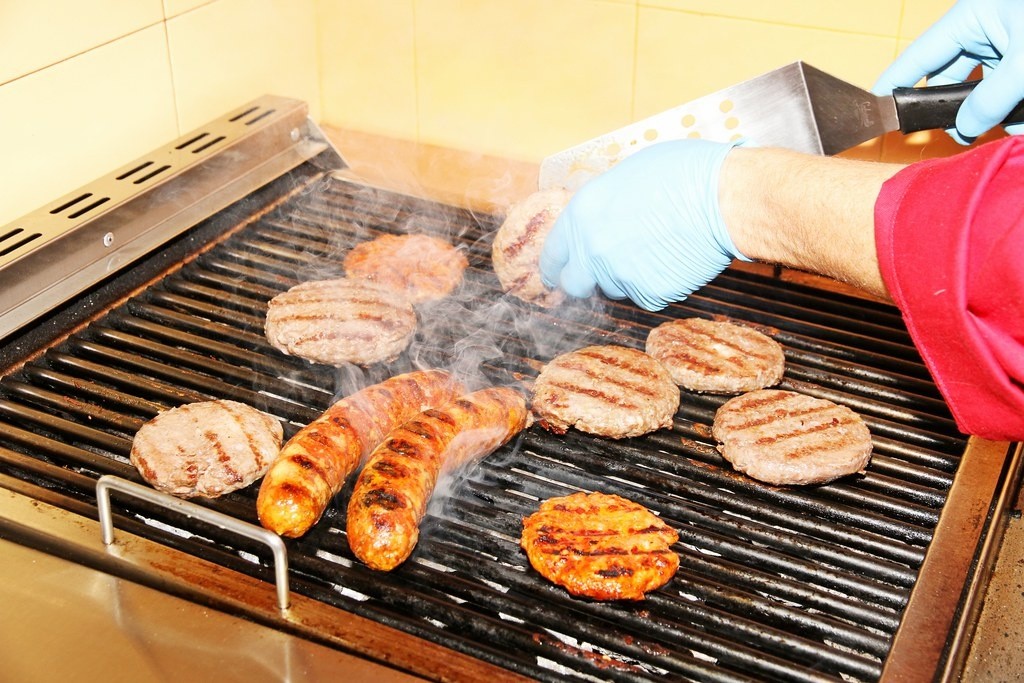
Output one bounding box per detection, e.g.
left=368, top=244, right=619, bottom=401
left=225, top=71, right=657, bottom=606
left=539, top=139, right=759, bottom=312
left=870, top=0, right=1024, bottom=146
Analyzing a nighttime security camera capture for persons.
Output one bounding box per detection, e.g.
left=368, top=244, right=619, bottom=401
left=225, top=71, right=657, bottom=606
left=539, top=0, right=1022, bottom=441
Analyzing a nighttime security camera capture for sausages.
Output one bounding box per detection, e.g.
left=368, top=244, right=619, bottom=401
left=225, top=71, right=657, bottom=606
left=346, top=386, right=528, bottom=571
left=255, top=369, right=469, bottom=539
